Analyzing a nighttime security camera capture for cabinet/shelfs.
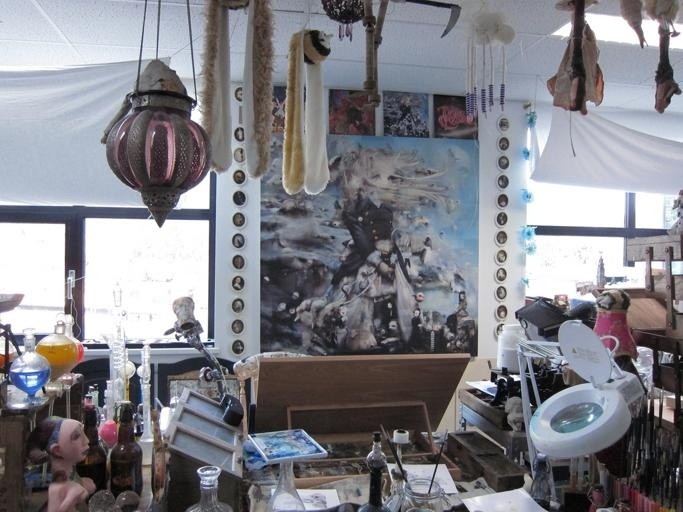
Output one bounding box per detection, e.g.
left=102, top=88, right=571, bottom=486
left=1, top=375, right=84, bottom=511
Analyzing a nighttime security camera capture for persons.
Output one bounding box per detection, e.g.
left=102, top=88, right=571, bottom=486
left=325, top=147, right=423, bottom=349
left=26, top=416, right=96, bottom=512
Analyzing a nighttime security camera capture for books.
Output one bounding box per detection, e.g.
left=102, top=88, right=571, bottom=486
left=248, top=429, right=328, bottom=464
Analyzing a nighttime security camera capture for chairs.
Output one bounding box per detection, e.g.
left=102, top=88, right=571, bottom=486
left=65, top=357, right=251, bottom=432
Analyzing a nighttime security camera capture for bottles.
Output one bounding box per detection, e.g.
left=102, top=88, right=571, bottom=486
left=10, top=321, right=85, bottom=400
left=75, top=403, right=142, bottom=503
left=186, top=465, right=234, bottom=512
left=365, top=431, right=387, bottom=507
left=264, top=460, right=307, bottom=512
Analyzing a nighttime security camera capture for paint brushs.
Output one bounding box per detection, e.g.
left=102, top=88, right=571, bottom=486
left=627, top=382, right=683, bottom=511
left=380, top=422, right=448, bottom=506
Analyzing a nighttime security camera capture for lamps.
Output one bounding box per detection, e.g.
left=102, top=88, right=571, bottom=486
left=101, top=0, right=212, bottom=226
